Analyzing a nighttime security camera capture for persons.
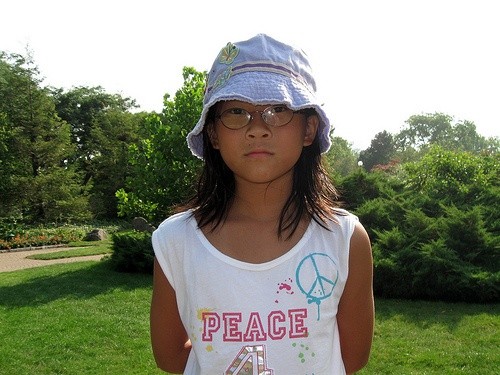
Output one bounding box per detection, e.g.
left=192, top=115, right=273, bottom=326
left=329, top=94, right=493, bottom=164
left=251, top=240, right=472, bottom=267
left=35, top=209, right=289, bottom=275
left=149, top=33, right=376, bottom=375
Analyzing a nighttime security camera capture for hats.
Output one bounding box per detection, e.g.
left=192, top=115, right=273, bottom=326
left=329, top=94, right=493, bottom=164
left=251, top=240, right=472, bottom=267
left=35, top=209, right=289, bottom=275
left=185, top=32, right=332, bottom=162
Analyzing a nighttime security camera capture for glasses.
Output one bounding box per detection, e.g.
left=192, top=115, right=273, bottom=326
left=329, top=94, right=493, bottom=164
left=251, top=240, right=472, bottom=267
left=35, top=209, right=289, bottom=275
left=214, top=104, right=306, bottom=130
